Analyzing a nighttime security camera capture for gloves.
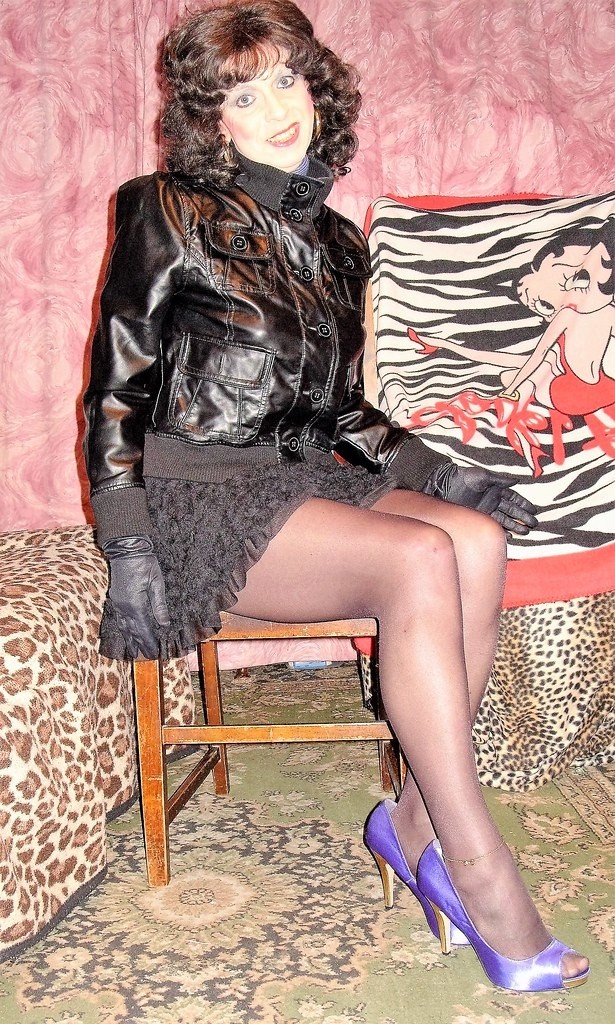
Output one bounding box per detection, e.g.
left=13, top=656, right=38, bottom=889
left=103, top=533, right=173, bottom=660
left=423, top=460, right=539, bottom=542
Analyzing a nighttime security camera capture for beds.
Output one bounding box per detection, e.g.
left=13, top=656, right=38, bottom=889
left=0, top=523, right=198, bottom=959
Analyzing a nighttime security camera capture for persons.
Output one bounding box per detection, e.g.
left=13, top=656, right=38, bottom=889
left=78, top=0, right=590, bottom=991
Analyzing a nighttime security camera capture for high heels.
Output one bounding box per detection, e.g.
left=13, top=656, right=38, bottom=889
left=363, top=798, right=471, bottom=945
left=414, top=838, right=592, bottom=992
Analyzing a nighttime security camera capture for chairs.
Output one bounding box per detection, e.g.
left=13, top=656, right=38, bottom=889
left=132, top=277, right=410, bottom=888
left=359, top=189, right=615, bottom=802
